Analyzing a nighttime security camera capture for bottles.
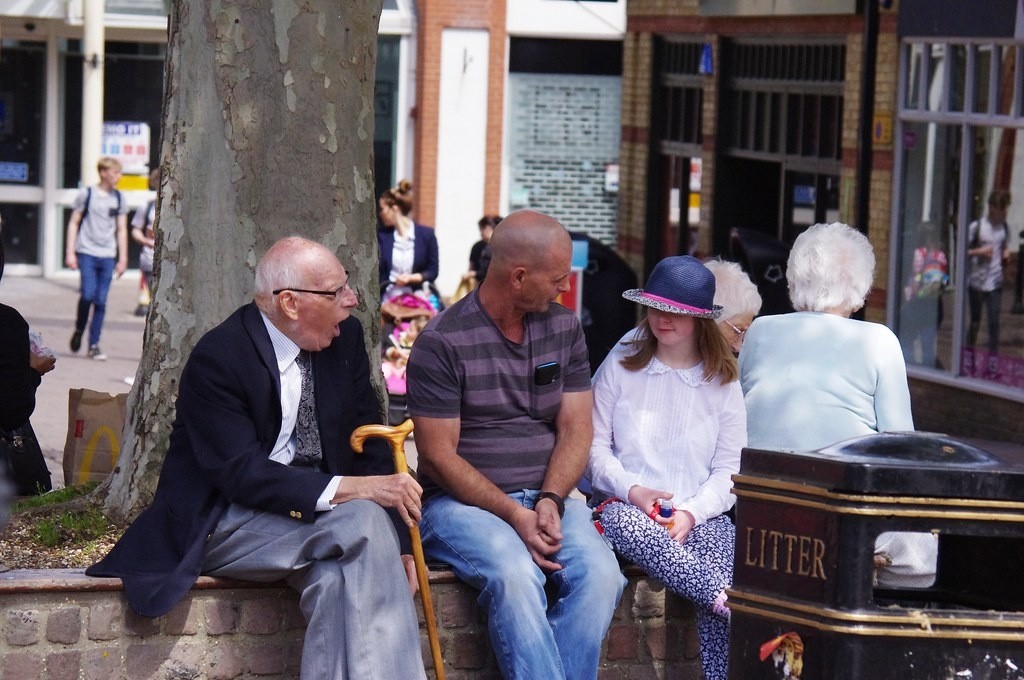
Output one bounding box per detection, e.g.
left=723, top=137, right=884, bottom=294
left=658, top=499, right=674, bottom=530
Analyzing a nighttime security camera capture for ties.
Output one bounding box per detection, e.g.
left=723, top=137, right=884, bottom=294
left=289, top=349, right=322, bottom=471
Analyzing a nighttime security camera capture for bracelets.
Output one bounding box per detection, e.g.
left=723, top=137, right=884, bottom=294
left=533, top=492, right=565, bottom=519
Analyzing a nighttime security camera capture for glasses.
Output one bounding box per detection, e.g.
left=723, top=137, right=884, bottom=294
left=722, top=317, right=746, bottom=341
left=273, top=271, right=350, bottom=303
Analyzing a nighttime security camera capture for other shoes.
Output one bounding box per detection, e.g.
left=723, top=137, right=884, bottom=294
left=70, top=332, right=82, bottom=350
left=88, top=346, right=105, bottom=360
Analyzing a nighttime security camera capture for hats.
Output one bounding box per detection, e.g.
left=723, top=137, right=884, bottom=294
left=622, top=256, right=725, bottom=319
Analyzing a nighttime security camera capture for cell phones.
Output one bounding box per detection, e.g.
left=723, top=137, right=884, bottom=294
left=535, top=361, right=562, bottom=386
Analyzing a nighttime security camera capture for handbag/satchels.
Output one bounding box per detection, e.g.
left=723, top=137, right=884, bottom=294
left=61, top=389, right=131, bottom=486
left=4, top=420, right=52, bottom=495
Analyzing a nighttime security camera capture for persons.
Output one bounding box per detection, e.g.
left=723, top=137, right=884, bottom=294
left=738, top=224, right=942, bottom=589
left=65, top=158, right=129, bottom=360
left=405, top=209, right=629, bottom=680
left=130, top=170, right=159, bottom=295
left=908, top=191, right=1009, bottom=369
left=85, top=236, right=429, bottom=680
left=703, top=258, right=762, bottom=355
left=377, top=179, right=503, bottom=368
left=0, top=238, right=56, bottom=498
left=586, top=255, right=748, bottom=680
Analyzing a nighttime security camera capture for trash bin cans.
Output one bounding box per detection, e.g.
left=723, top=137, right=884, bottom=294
left=728, top=430, right=1024, bottom=680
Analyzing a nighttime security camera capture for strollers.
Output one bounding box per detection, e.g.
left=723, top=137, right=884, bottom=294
left=379, top=279, right=448, bottom=426
